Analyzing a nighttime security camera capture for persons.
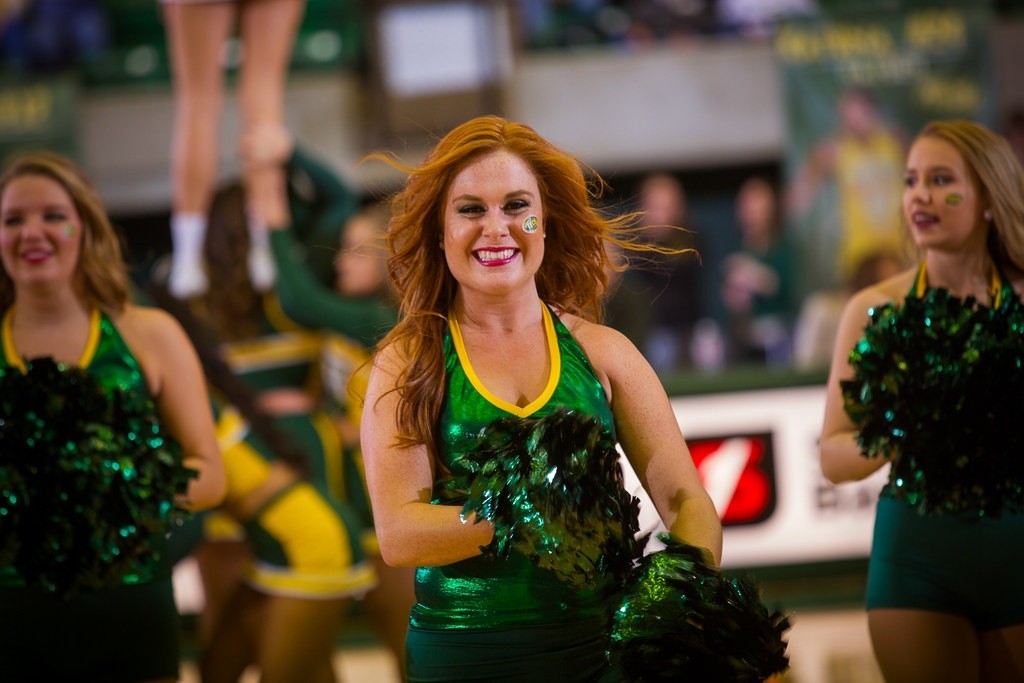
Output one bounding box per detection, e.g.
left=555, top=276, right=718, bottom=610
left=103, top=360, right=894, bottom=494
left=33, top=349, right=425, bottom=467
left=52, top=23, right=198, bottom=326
left=820, top=119, right=1024, bottom=682
left=587, top=0, right=798, bottom=375
left=359, top=114, right=786, bottom=682
left=782, top=89, right=918, bottom=373
left=0, top=0, right=403, bottom=682
